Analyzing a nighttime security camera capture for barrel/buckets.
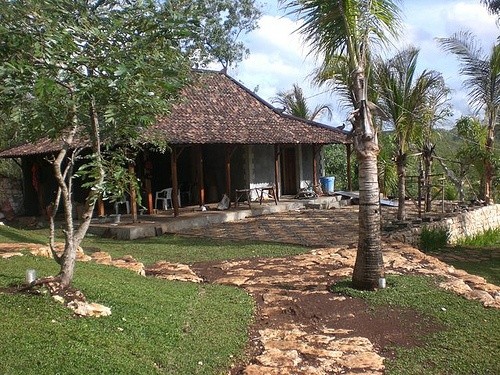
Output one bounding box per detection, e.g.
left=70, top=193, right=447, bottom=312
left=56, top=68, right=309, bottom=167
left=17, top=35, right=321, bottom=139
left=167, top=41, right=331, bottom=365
left=109, top=214, right=121, bottom=223
left=319, top=176, right=334, bottom=194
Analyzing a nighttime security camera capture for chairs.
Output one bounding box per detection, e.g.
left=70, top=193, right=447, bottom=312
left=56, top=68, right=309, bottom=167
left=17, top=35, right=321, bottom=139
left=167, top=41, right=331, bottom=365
left=155, top=187, right=174, bottom=210
left=116, top=196, right=131, bottom=213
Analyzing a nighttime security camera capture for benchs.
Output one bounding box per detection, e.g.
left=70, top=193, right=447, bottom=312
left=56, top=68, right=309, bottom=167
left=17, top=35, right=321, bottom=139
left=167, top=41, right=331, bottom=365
left=233, top=185, right=278, bottom=210
